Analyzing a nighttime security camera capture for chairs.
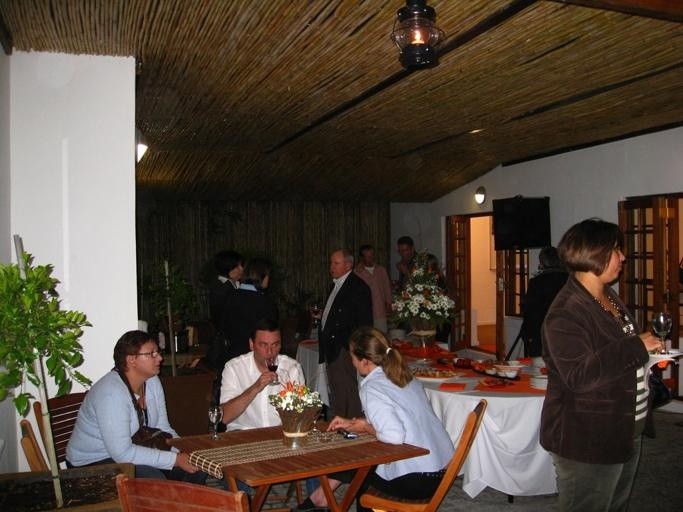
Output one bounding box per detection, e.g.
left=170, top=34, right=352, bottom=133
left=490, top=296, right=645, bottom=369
left=115, top=473, right=250, bottom=512
left=20, top=417, right=48, bottom=472
left=33, top=390, right=90, bottom=473
left=360, top=399, right=487, bottom=512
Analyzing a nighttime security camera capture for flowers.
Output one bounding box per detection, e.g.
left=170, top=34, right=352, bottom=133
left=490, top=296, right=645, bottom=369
left=268, top=380, right=323, bottom=408
left=388, top=246, right=456, bottom=331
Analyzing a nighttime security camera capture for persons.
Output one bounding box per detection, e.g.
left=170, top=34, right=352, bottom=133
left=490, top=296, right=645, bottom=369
left=65, top=330, right=208, bottom=485
left=310, top=249, right=373, bottom=419
left=520, top=247, right=570, bottom=358
left=291, top=325, right=455, bottom=512
left=540, top=217, right=664, bottom=512
left=209, top=249, right=244, bottom=322
left=427, top=255, right=453, bottom=343
left=219, top=317, right=321, bottom=497
left=353, top=244, right=392, bottom=335
left=397, top=237, right=419, bottom=293
left=209, top=262, right=279, bottom=435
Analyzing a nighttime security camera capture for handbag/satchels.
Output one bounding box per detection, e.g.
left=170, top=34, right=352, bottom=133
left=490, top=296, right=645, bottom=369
left=111, top=366, right=172, bottom=452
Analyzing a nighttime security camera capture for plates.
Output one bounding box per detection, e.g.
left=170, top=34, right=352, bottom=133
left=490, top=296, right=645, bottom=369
left=505, top=371, right=517, bottom=378
left=531, top=385, right=547, bottom=391
left=649, top=350, right=681, bottom=360
left=494, top=364, right=526, bottom=371
left=416, top=373, right=459, bottom=384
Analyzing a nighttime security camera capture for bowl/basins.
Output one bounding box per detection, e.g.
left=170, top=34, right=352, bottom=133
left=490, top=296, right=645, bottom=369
left=508, top=361, right=521, bottom=366
left=497, top=370, right=505, bottom=377
left=485, top=368, right=496, bottom=375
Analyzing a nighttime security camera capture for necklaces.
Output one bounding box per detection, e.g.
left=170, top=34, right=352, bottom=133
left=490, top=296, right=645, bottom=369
left=591, top=294, right=634, bottom=335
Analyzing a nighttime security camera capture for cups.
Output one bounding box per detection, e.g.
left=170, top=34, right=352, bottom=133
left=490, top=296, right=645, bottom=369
left=536, top=375, right=548, bottom=387
left=208, top=406, right=223, bottom=441
left=652, top=312, right=672, bottom=352
left=312, top=304, right=320, bottom=330
left=265, top=356, right=281, bottom=386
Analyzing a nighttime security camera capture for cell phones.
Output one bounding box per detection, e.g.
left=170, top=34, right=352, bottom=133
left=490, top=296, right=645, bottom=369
left=341, top=430, right=359, bottom=439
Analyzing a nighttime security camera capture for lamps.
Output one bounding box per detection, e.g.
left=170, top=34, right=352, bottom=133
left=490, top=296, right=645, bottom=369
left=390, top=0, right=446, bottom=71
left=475, top=187, right=487, bottom=208
left=135, top=126, right=148, bottom=163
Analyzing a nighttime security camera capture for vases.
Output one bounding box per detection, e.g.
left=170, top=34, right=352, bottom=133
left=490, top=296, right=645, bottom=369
left=276, top=407, right=323, bottom=448
left=409, top=331, right=437, bottom=364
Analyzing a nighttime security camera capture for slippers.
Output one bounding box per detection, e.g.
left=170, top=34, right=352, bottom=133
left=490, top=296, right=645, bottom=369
left=290, top=497, right=331, bottom=512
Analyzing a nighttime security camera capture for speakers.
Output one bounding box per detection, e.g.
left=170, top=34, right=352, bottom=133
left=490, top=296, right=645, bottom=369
left=491, top=194, right=553, bottom=250
